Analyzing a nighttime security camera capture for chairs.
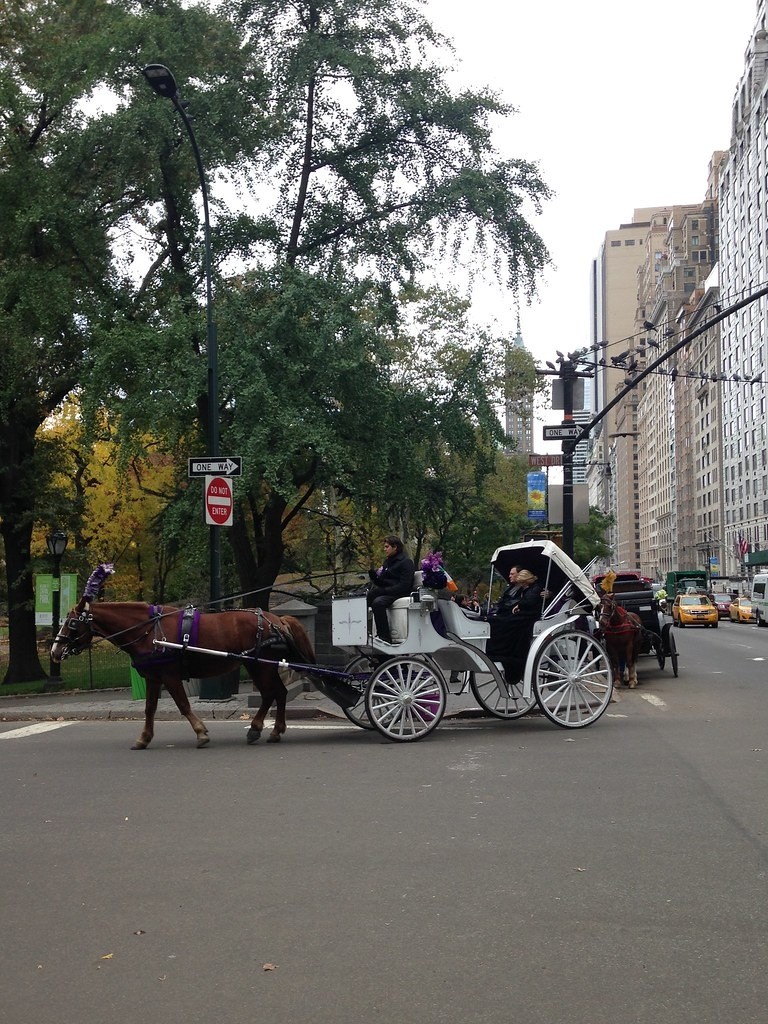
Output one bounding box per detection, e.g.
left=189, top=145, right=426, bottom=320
left=370, top=570, right=424, bottom=642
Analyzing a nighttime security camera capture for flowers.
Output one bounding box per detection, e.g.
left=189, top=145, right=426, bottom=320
left=654, top=589, right=668, bottom=599
left=377, top=566, right=384, bottom=575
left=419, top=551, right=444, bottom=579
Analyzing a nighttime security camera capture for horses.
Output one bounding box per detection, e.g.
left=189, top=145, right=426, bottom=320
left=49, top=595, right=314, bottom=750
left=599, top=592, right=642, bottom=689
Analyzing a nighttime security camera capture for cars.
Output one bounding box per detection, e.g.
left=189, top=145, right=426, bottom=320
left=651, top=583, right=666, bottom=613
left=710, top=593, right=756, bottom=625
left=671, top=595, right=719, bottom=628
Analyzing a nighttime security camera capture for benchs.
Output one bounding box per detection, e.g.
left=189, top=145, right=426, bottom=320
left=439, top=569, right=573, bottom=656
left=597, top=579, right=654, bottom=606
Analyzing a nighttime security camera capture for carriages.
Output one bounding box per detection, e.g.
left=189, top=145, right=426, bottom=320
left=48, top=539, right=613, bottom=752
left=592, top=571, right=679, bottom=689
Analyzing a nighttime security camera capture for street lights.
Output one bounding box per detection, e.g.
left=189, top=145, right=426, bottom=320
left=140, top=60, right=224, bottom=710
left=45, top=517, right=68, bottom=693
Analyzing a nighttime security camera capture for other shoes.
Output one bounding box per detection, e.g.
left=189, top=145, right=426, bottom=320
left=383, top=638, right=392, bottom=645
left=450, top=678, right=462, bottom=683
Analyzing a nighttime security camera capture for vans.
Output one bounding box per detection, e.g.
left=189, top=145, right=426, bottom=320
left=750, top=569, right=768, bottom=626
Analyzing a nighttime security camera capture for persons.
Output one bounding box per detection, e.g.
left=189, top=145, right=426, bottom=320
left=448, top=591, right=480, bottom=613
left=655, top=586, right=668, bottom=600
left=450, top=594, right=481, bottom=683
left=496, top=564, right=553, bottom=616
left=366, top=535, right=414, bottom=642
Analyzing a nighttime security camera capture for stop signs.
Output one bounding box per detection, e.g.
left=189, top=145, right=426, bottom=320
left=205, top=474, right=238, bottom=526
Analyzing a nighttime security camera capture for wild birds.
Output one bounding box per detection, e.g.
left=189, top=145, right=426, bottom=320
left=546, top=319, right=763, bottom=386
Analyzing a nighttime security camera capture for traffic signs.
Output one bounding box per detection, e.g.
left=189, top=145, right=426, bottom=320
left=543, top=423, right=591, bottom=440
left=187, top=457, right=242, bottom=477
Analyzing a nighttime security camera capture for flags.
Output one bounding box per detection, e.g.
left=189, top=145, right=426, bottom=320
left=740, top=539, right=747, bottom=554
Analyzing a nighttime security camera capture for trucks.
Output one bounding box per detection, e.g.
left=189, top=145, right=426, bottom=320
left=666, top=570, right=713, bottom=616
